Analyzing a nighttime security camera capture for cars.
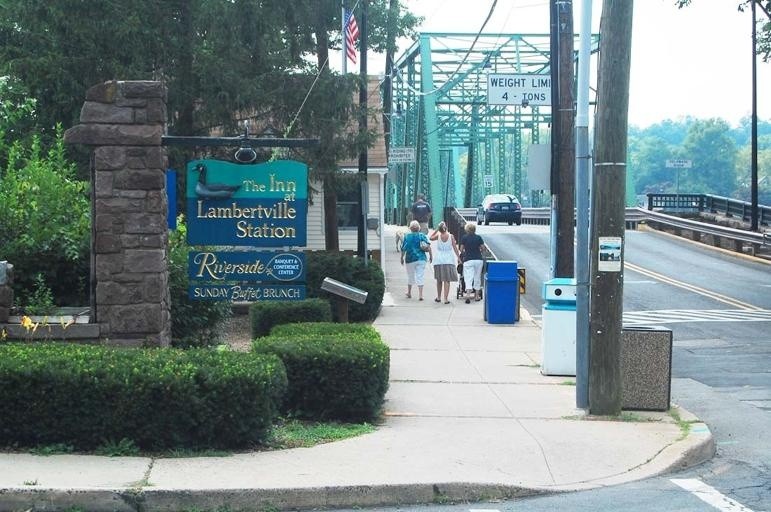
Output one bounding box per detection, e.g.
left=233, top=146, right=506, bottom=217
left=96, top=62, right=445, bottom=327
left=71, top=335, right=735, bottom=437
left=475, top=193, right=522, bottom=226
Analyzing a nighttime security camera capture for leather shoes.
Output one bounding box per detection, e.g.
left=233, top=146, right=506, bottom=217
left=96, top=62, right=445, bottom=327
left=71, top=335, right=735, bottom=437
left=404, top=290, right=482, bottom=305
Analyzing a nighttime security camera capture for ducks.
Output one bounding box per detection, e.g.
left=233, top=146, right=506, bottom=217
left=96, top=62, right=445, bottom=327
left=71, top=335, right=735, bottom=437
left=192, top=164, right=241, bottom=199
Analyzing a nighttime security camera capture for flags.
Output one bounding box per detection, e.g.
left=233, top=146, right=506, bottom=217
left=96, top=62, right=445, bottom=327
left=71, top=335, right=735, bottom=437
left=345, top=10, right=359, bottom=64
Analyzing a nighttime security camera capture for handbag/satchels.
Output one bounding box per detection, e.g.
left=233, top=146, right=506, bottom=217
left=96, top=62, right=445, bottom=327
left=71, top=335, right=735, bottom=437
left=419, top=231, right=431, bottom=252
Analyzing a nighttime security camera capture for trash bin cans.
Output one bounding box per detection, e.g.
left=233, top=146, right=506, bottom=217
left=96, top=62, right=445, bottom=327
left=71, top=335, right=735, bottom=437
left=483, top=260, right=521, bottom=324
left=541, top=277, right=578, bottom=378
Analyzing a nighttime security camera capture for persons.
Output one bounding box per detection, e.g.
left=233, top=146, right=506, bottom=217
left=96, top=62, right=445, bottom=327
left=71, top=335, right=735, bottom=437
left=409, top=194, right=433, bottom=236
left=401, top=220, right=433, bottom=300
left=458, top=223, right=488, bottom=303
left=430, top=221, right=460, bottom=304
